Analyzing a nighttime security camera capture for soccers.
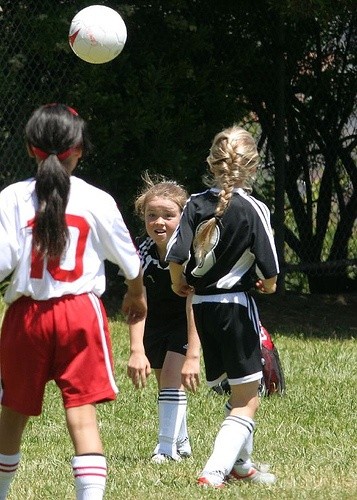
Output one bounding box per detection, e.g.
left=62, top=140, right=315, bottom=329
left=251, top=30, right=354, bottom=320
left=68, top=4, right=127, bottom=65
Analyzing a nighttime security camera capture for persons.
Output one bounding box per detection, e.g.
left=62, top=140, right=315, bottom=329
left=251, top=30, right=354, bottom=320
left=128, top=175, right=203, bottom=466
left=165, top=126, right=280, bottom=488
left=211, top=322, right=286, bottom=417
left=0, top=105, right=146, bottom=500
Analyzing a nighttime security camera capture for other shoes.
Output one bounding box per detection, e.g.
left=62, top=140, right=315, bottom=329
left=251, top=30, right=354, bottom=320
left=197, top=477, right=226, bottom=489
left=228, top=467, right=275, bottom=486
left=152, top=448, right=182, bottom=469
left=176, top=436, right=191, bottom=458
